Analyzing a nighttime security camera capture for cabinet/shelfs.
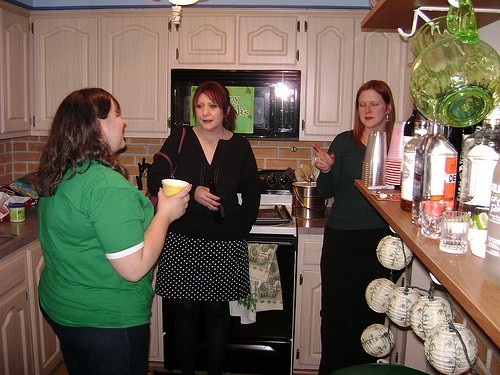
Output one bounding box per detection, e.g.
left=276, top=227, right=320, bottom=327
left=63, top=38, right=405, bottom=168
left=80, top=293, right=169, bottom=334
left=0, top=6, right=32, bottom=137
left=32, top=15, right=168, bottom=135
left=294, top=234, right=324, bottom=370
left=0, top=251, right=38, bottom=375
left=26, top=242, right=62, bottom=375
left=354, top=0, right=499, bottom=349
left=177, top=14, right=300, bottom=66
left=303, top=13, right=411, bottom=137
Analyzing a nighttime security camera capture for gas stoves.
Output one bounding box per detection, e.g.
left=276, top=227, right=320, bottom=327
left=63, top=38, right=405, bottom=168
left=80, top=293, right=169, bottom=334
left=248, top=166, right=297, bottom=235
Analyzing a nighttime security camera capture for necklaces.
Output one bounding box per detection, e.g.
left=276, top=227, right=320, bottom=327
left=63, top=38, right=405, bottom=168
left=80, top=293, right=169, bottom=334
left=199, top=131, right=214, bottom=152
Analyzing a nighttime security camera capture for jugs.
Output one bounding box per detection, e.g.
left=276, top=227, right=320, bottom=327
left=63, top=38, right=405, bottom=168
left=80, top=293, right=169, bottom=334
left=412, top=0, right=500, bottom=127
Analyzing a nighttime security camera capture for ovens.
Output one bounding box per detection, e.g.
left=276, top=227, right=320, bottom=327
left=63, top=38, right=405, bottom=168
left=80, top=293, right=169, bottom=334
left=185, top=234, right=295, bottom=375
left=169, top=68, right=301, bottom=140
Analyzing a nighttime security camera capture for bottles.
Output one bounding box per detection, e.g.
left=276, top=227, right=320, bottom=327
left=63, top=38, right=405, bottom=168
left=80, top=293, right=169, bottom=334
left=209, top=178, right=226, bottom=225
left=401, top=99, right=500, bottom=283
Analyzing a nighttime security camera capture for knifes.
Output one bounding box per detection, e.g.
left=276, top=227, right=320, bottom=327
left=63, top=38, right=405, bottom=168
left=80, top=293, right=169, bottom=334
left=136, top=157, right=150, bottom=189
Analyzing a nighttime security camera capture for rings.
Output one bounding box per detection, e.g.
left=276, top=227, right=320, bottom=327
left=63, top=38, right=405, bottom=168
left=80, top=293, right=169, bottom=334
left=314, top=156, right=318, bottom=161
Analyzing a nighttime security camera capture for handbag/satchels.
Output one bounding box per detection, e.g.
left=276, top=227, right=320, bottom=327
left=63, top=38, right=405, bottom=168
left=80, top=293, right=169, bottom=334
left=146, top=128, right=187, bottom=214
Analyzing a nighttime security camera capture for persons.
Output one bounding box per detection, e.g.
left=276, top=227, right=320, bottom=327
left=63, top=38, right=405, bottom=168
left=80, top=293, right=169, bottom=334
left=36, top=87, right=193, bottom=375
left=147, top=82, right=261, bottom=375
left=312, top=80, right=406, bottom=375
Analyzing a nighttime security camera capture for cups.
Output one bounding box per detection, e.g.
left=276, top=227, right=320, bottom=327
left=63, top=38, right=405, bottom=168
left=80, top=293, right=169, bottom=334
left=362, top=122, right=406, bottom=188
left=161, top=179, right=190, bottom=197
left=438, top=211, right=471, bottom=254
left=420, top=200, right=447, bottom=239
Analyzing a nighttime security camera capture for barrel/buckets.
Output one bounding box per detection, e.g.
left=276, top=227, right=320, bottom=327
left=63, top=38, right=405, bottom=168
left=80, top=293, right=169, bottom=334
left=291, top=182, right=330, bottom=218
